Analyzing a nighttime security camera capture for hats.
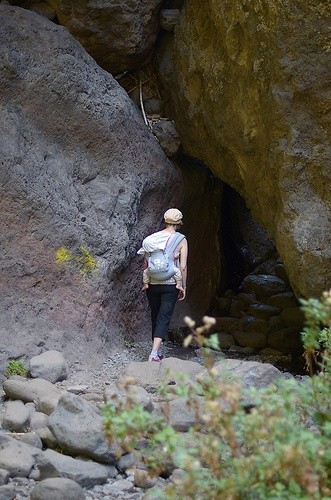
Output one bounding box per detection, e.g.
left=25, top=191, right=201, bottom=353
left=164, top=208, right=183, bottom=225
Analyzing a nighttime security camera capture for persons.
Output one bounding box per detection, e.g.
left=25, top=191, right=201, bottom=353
left=142, top=236, right=184, bottom=292
left=142, top=208, right=188, bottom=362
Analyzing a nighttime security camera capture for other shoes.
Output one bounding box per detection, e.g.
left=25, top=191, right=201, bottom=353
left=156, top=352, right=166, bottom=360
left=148, top=355, right=161, bottom=362
